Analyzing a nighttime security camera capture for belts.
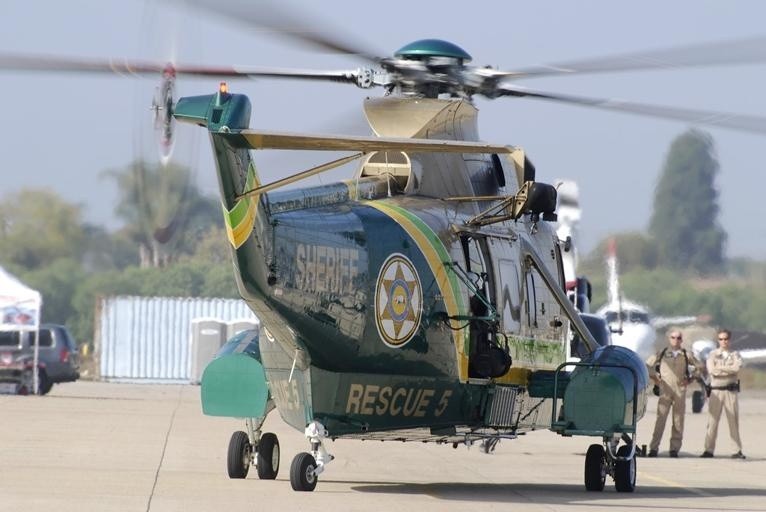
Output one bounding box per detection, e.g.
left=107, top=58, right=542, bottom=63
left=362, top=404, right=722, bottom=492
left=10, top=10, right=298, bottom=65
left=711, top=385, right=737, bottom=391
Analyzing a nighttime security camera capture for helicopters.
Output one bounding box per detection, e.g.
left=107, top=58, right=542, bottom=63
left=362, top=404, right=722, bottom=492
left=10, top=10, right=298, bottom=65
left=0, top=0, right=766, bottom=496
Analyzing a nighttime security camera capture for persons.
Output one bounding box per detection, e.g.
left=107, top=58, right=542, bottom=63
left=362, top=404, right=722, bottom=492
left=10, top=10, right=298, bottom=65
left=645, top=326, right=701, bottom=457
left=699, top=329, right=747, bottom=458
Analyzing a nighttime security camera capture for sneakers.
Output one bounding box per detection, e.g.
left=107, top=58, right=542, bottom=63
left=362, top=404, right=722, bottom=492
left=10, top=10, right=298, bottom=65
left=700, top=452, right=713, bottom=457
left=670, top=451, right=676, bottom=456
left=732, top=452, right=745, bottom=458
left=648, top=450, right=657, bottom=456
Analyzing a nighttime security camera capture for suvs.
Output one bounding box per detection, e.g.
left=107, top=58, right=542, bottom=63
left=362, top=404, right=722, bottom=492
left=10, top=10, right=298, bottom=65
left=0, top=323, right=80, bottom=395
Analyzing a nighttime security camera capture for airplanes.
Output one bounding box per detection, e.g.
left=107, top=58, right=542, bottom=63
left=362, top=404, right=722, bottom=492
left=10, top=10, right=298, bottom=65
left=590, top=235, right=766, bottom=381
